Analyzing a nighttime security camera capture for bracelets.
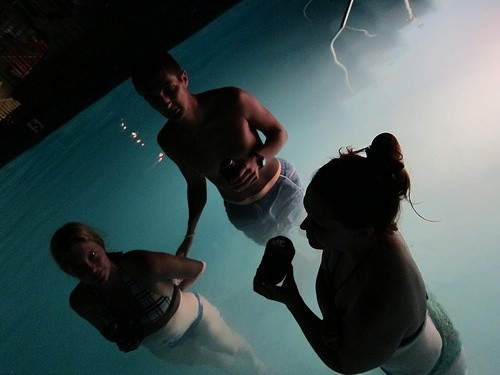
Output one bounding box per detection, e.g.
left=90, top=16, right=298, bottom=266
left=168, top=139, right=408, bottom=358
left=250, top=151, right=267, bottom=170
left=185, top=233, right=194, bottom=238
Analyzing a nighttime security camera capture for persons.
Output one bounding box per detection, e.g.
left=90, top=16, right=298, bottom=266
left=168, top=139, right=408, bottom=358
left=48, top=222, right=266, bottom=375
left=128, top=47, right=306, bottom=259
left=253, top=133, right=468, bottom=375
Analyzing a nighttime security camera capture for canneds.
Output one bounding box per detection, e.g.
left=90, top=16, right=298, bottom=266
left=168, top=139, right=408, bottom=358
left=258, top=236, right=295, bottom=285
left=219, top=158, right=238, bottom=183
left=105, top=321, right=130, bottom=343
